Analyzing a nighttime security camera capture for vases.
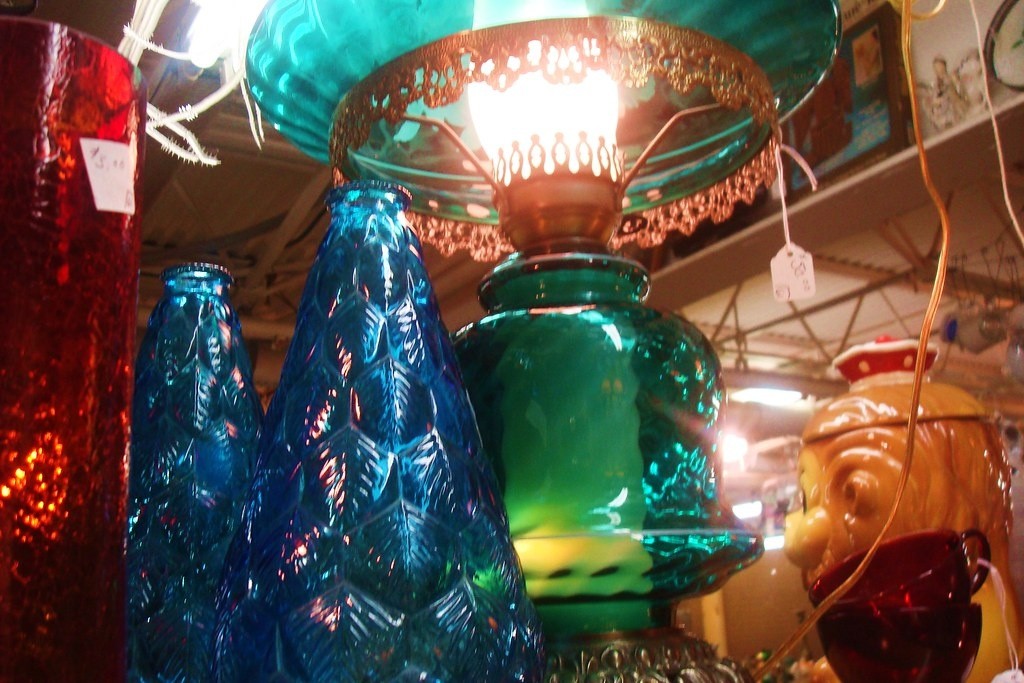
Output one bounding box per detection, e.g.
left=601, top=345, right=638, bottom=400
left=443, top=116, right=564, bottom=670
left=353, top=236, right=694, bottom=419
left=0, top=13, right=545, bottom=683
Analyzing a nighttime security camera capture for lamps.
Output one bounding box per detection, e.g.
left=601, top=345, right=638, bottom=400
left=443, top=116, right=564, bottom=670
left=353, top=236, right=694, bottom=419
left=242, top=0, right=844, bottom=683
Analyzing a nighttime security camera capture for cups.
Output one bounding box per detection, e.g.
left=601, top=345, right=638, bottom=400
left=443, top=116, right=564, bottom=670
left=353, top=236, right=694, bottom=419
left=0, top=14, right=147, bottom=683
left=811, top=529, right=993, bottom=609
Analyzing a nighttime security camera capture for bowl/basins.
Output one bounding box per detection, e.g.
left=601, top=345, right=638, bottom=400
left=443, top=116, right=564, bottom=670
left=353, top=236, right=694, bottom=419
left=816, top=601, right=983, bottom=683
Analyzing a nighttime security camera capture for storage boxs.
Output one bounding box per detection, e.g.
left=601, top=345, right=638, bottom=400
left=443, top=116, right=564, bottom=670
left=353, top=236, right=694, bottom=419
left=784, top=0, right=916, bottom=202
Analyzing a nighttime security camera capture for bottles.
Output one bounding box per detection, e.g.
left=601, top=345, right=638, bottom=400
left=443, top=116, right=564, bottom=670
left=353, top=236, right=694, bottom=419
left=125, top=262, right=266, bottom=683
left=211, top=180, right=549, bottom=683
